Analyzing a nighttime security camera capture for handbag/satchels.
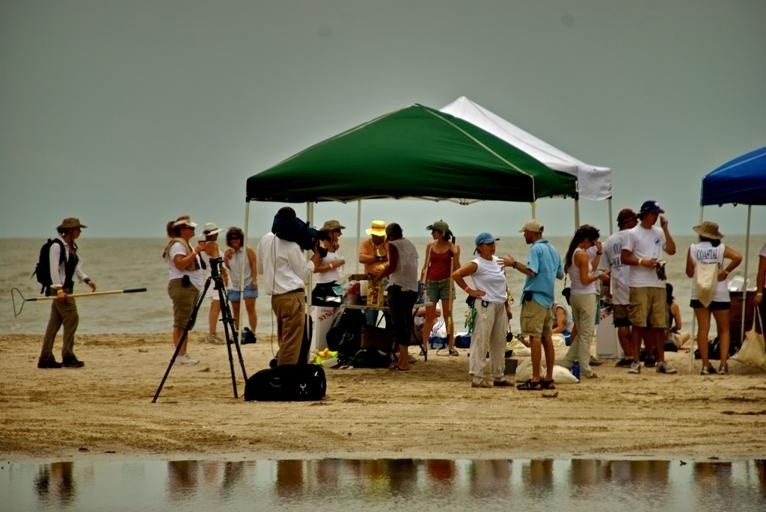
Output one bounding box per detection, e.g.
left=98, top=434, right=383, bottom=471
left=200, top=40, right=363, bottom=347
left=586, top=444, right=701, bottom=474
left=729, top=330, right=766, bottom=372
left=244, top=363, right=327, bottom=402
left=326, top=308, right=365, bottom=354
left=694, top=335, right=742, bottom=359
left=229, top=327, right=256, bottom=344
left=694, top=261, right=719, bottom=308
left=562, top=288, right=571, bottom=305
left=330, top=349, right=397, bottom=370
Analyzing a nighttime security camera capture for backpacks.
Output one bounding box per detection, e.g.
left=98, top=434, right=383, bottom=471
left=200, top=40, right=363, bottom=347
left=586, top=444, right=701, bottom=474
left=31, top=238, right=65, bottom=296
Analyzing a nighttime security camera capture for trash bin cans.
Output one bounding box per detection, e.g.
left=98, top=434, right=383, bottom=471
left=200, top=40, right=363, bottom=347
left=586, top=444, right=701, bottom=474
left=728, top=289, right=761, bottom=354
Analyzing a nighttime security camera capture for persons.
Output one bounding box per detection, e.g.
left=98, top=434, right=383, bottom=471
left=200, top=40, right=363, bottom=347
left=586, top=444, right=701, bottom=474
left=752, top=244, right=766, bottom=343
left=600, top=209, right=657, bottom=368
left=358, top=220, right=392, bottom=331
left=560, top=224, right=611, bottom=379
left=35, top=217, right=96, bottom=368
left=418, top=218, right=462, bottom=357
left=310, top=220, right=348, bottom=352
left=550, top=300, right=575, bottom=345
left=257, top=207, right=331, bottom=366
left=621, top=199, right=678, bottom=374
left=496, top=219, right=565, bottom=391
left=686, top=220, right=743, bottom=375
left=662, top=283, right=690, bottom=352
left=161, top=215, right=208, bottom=366
left=372, top=223, right=418, bottom=371
left=223, top=226, right=260, bottom=345
left=451, top=233, right=515, bottom=388
left=200, top=222, right=234, bottom=345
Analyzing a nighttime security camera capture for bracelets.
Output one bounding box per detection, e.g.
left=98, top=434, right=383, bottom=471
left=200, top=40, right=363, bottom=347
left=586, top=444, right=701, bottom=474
left=756, top=292, right=764, bottom=296
left=596, top=250, right=603, bottom=256
left=193, top=250, right=198, bottom=256
left=84, top=278, right=90, bottom=284
left=723, top=269, right=731, bottom=276
left=328, top=262, right=335, bottom=270
left=595, top=271, right=600, bottom=278
left=511, top=261, right=518, bottom=268
left=637, top=258, right=644, bottom=265
left=464, top=285, right=470, bottom=291
left=57, top=289, right=64, bottom=293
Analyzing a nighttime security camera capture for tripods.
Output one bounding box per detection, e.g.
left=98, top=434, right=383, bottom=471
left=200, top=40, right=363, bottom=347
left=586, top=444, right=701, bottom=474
left=153, top=257, right=248, bottom=403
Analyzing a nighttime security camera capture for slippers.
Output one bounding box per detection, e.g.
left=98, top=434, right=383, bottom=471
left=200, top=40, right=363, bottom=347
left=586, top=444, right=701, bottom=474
left=390, top=364, right=410, bottom=371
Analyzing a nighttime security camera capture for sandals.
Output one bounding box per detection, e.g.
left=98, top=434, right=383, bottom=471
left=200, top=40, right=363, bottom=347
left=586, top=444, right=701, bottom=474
left=541, top=379, right=555, bottom=389
left=517, top=379, right=543, bottom=391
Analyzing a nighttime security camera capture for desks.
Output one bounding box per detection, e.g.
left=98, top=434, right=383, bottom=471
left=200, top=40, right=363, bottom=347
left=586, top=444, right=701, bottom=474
left=340, top=305, right=432, bottom=369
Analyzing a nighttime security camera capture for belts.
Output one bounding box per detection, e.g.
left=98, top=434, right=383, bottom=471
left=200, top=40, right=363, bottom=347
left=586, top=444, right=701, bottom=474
left=427, top=277, right=449, bottom=283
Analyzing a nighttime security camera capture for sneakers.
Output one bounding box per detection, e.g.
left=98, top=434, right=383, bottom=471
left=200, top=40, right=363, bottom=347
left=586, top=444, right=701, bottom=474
left=701, top=364, right=717, bottom=375
left=449, top=346, right=459, bottom=356
left=494, top=380, right=514, bottom=386
left=654, top=361, right=677, bottom=374
left=471, top=379, right=493, bottom=388
left=627, top=360, right=641, bottom=374
left=718, top=365, right=728, bottom=374
left=615, top=358, right=634, bottom=367
left=420, top=344, right=427, bottom=356
left=645, top=356, right=656, bottom=366
left=38, top=360, right=62, bottom=368
left=63, top=360, right=84, bottom=368
left=208, top=335, right=225, bottom=344
left=590, top=356, right=604, bottom=366
left=175, top=354, right=200, bottom=364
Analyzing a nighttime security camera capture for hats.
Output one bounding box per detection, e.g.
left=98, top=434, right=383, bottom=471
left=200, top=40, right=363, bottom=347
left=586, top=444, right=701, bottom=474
left=321, top=221, right=346, bottom=231
left=426, top=219, right=449, bottom=232
left=518, top=219, right=545, bottom=233
left=200, top=223, right=224, bottom=237
left=727, top=275, right=758, bottom=292
left=639, top=201, right=665, bottom=213
left=365, top=220, right=387, bottom=237
left=616, top=208, right=640, bottom=222
left=173, top=218, right=197, bottom=228
left=474, top=233, right=500, bottom=255
left=61, top=218, right=87, bottom=229
left=692, top=221, right=724, bottom=240
left=385, top=224, right=401, bottom=243
